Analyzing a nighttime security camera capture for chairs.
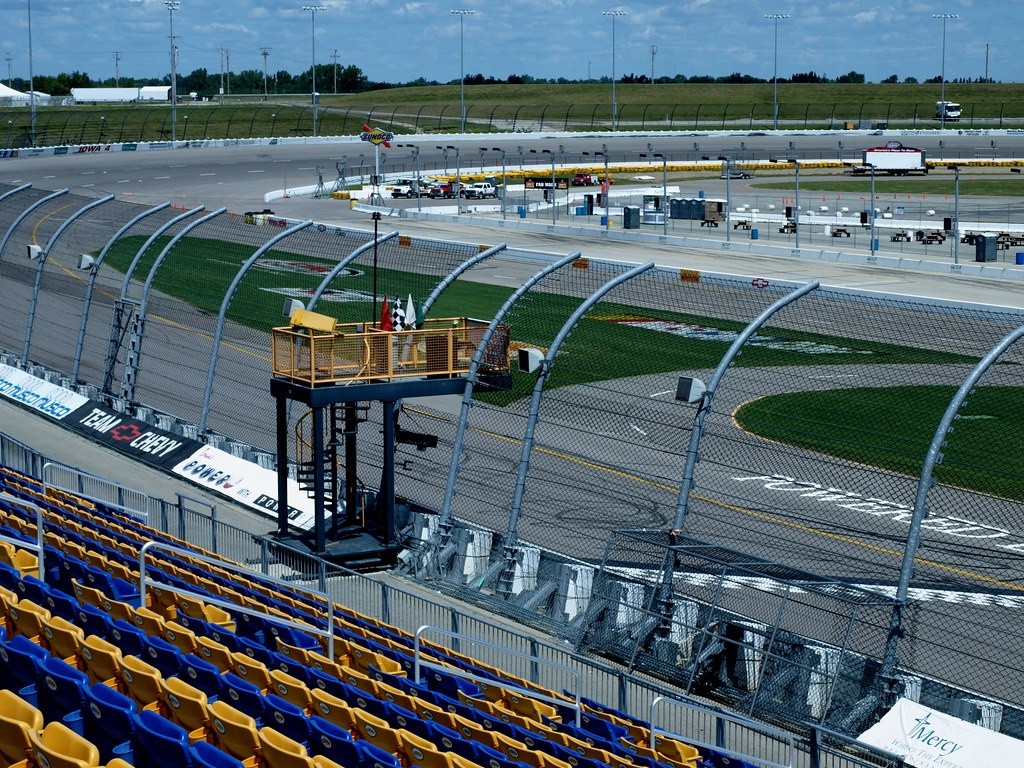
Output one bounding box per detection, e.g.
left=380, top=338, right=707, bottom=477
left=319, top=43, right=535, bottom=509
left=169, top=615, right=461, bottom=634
left=0, top=463, right=764, bottom=768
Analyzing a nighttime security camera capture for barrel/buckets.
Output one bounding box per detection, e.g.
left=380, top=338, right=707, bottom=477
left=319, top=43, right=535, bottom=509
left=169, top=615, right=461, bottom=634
left=865, top=210, right=871, bottom=217
left=601, top=217, right=607, bottom=225
left=871, top=239, right=878, bottom=250
left=873, top=210, right=877, bottom=218
left=751, top=229, right=758, bottom=239
left=699, top=191, right=705, bottom=198
left=576, top=207, right=585, bottom=215
left=1016, top=252, right=1024, bottom=265
left=518, top=206, right=523, bottom=213
left=520, top=210, right=526, bottom=218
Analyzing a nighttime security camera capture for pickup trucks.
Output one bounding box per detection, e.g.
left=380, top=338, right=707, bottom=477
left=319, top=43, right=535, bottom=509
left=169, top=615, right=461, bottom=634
left=430, top=181, right=465, bottom=201
left=465, top=183, right=497, bottom=199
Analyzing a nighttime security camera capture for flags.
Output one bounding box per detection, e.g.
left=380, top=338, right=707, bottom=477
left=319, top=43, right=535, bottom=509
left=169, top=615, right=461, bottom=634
left=381, top=292, right=424, bottom=331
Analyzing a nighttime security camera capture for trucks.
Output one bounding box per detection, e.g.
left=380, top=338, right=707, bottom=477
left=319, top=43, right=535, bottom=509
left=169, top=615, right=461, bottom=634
left=935, top=101, right=962, bottom=121
left=391, top=177, right=437, bottom=198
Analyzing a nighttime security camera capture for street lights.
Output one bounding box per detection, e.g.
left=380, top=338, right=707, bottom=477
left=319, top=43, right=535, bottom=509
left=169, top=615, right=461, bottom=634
left=302, top=6, right=328, bottom=136
left=492, top=148, right=507, bottom=220
left=931, top=13, right=959, bottom=129
left=785, top=158, right=800, bottom=249
left=450, top=8, right=476, bottom=132
left=406, top=144, right=422, bottom=212
left=942, top=166, right=962, bottom=265
left=763, top=14, right=791, bottom=129
left=653, top=153, right=670, bottom=235
left=594, top=152, right=610, bottom=231
left=163, top=0, right=181, bottom=141
left=447, top=145, right=462, bottom=215
left=540, top=148, right=556, bottom=225
left=436, top=145, right=448, bottom=175
left=860, top=162, right=874, bottom=257
left=397, top=144, right=416, bottom=178
left=717, top=156, right=732, bottom=243
left=530, top=149, right=539, bottom=165
left=479, top=146, right=488, bottom=173
left=602, top=10, right=628, bottom=131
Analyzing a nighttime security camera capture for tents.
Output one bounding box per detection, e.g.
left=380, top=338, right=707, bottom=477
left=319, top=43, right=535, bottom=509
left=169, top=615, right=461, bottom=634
left=0, top=83, right=35, bottom=106
